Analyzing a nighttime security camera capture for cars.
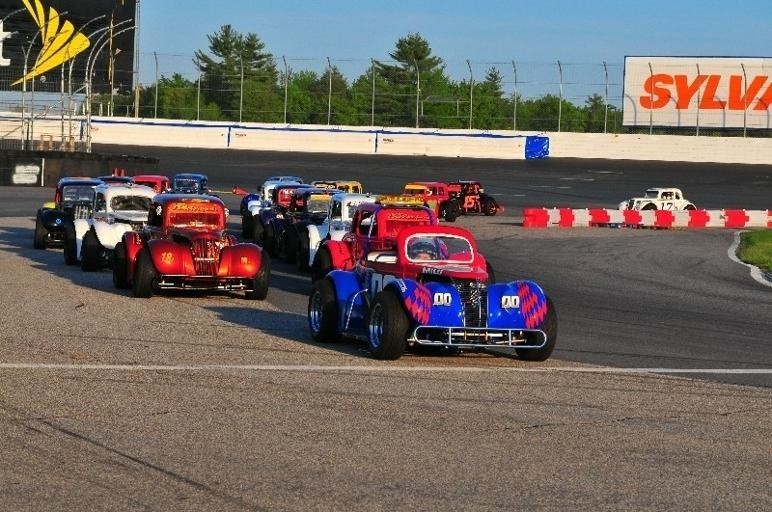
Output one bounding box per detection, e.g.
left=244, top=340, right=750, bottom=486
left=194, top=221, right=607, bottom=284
left=34, top=176, right=103, bottom=250
left=447, top=178, right=504, bottom=218
left=131, top=173, right=170, bottom=193
left=239, top=177, right=378, bottom=267
left=616, top=187, right=698, bottom=212
left=311, top=203, right=440, bottom=281
left=112, top=194, right=271, bottom=303
left=305, top=204, right=559, bottom=363
left=400, top=181, right=456, bottom=218
left=62, top=181, right=159, bottom=271
left=164, top=173, right=220, bottom=198
left=98, top=175, right=132, bottom=185
